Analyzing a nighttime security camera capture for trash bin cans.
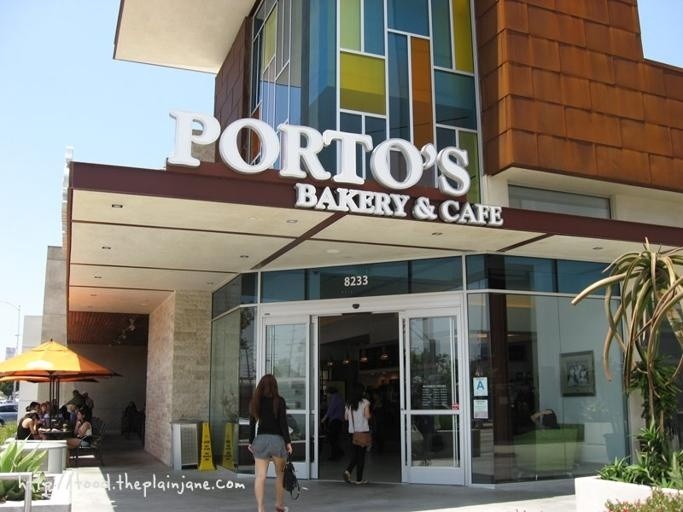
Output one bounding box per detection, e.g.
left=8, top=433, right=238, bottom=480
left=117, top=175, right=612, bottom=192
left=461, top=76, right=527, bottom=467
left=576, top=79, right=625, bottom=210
left=171, top=422, right=198, bottom=471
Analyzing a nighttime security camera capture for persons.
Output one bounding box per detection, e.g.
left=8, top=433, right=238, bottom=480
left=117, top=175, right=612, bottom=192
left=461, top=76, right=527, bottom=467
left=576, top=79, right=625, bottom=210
left=15, top=389, right=95, bottom=449
left=320, top=381, right=374, bottom=486
left=248, top=373, right=300, bottom=512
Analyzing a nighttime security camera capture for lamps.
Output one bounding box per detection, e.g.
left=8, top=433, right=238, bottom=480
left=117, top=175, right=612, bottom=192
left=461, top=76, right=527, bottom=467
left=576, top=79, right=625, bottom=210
left=342, top=353, right=350, bottom=366
left=379, top=346, right=391, bottom=360
left=359, top=347, right=369, bottom=363
left=327, top=354, right=334, bottom=367
left=127, top=319, right=136, bottom=332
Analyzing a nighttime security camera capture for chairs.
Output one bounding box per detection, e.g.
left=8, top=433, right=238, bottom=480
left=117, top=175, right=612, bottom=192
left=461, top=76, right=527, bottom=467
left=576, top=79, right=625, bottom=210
left=513, top=429, right=579, bottom=479
left=15, top=416, right=103, bottom=467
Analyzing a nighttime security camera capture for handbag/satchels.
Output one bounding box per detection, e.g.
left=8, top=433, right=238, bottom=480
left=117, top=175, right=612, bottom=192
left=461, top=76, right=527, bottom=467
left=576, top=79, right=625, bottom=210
left=283, top=447, right=302, bottom=499
left=352, top=431, right=372, bottom=449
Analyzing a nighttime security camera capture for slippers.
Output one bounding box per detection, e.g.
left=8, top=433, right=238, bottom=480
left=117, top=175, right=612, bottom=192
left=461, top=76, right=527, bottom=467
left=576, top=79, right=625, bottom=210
left=343, top=470, right=368, bottom=485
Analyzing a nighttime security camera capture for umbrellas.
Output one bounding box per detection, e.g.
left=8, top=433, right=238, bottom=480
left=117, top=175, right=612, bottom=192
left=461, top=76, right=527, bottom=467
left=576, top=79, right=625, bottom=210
left=0, top=338, right=124, bottom=430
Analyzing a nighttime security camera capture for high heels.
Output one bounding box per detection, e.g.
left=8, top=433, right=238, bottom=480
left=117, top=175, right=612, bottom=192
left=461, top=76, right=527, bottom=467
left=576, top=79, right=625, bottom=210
left=275, top=506, right=288, bottom=512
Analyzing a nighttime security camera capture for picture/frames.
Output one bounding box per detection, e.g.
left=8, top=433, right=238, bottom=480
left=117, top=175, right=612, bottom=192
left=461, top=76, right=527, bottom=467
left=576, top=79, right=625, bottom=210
left=558, top=350, right=596, bottom=397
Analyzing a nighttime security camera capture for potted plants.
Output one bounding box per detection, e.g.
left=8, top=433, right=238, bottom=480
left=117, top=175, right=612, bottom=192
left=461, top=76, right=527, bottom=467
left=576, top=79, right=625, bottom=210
left=1, top=439, right=73, bottom=512
left=574, top=238, right=683, bottom=510
